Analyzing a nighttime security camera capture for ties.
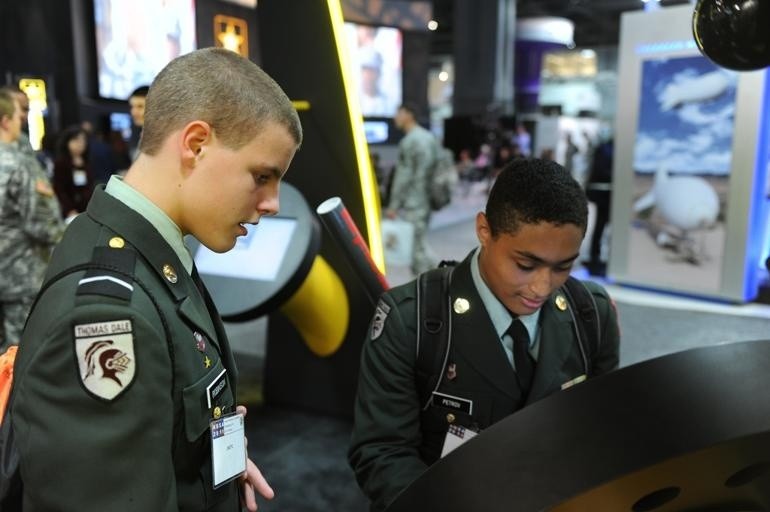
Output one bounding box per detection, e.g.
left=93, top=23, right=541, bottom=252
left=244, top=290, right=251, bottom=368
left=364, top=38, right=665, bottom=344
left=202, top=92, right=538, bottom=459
left=188, top=257, right=220, bottom=331
left=508, top=319, right=539, bottom=390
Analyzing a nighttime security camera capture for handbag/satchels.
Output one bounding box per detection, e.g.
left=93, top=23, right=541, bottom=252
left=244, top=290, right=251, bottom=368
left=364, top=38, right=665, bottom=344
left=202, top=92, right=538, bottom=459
left=380, top=212, right=416, bottom=268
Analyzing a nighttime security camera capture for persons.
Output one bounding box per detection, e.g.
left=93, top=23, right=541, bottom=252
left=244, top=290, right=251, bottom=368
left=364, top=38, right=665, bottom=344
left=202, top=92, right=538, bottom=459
left=43, top=127, right=100, bottom=221
left=475, top=142, right=494, bottom=180
left=454, top=144, right=476, bottom=180
left=348, top=156, right=622, bottom=510
left=126, top=86, right=151, bottom=130
left=0, top=87, right=69, bottom=358
left=370, top=153, right=383, bottom=187
left=491, top=114, right=522, bottom=170
left=585, top=137, right=612, bottom=268
left=355, top=46, right=394, bottom=115
left=0, top=46, right=307, bottom=511
left=386, top=100, right=460, bottom=279
left=512, top=121, right=533, bottom=158
left=2, top=83, right=63, bottom=224
left=566, top=134, right=578, bottom=172
left=97, top=114, right=131, bottom=176
left=344, top=22, right=382, bottom=58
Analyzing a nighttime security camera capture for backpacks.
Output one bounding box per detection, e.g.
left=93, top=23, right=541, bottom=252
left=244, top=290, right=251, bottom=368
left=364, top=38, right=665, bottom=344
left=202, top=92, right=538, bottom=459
left=425, top=141, right=459, bottom=210
